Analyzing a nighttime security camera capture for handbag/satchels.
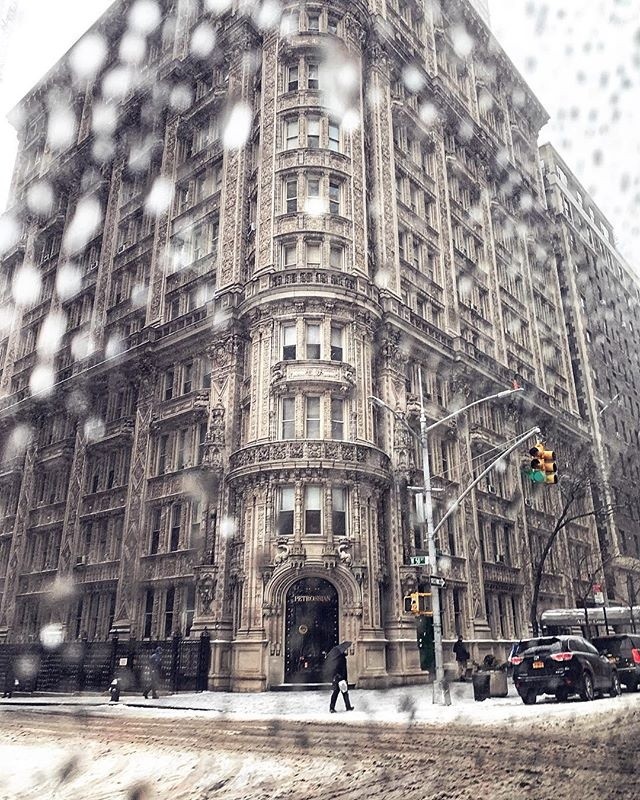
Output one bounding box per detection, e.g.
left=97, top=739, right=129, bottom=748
left=338, top=680, right=348, bottom=693
left=459, top=652, right=470, bottom=659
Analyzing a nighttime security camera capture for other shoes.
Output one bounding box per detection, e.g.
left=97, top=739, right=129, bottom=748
left=330, top=708, right=336, bottom=712
left=152, top=696, right=159, bottom=698
left=346, top=707, right=354, bottom=711
left=143, top=693, right=148, bottom=698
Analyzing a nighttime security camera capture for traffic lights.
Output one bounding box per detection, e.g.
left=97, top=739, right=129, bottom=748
left=403, top=595, right=419, bottom=614
left=543, top=450, right=558, bottom=484
left=528, top=444, right=546, bottom=483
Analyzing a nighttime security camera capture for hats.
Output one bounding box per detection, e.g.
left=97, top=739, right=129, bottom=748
left=156, top=647, right=165, bottom=652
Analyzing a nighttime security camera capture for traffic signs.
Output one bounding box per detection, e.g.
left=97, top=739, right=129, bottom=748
left=429, top=574, right=446, bottom=589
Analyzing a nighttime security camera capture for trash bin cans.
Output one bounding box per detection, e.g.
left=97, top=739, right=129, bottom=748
left=473, top=674, right=490, bottom=701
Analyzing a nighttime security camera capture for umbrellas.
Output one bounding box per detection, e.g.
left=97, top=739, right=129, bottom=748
left=324, top=641, right=352, bottom=666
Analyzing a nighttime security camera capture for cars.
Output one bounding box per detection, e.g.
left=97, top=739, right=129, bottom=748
left=505, top=633, right=622, bottom=704
left=590, top=632, right=640, bottom=692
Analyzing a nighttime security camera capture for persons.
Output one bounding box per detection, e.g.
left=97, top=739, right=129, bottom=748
left=330, top=653, right=354, bottom=713
left=143, top=649, right=163, bottom=699
left=2, top=664, right=16, bottom=698
left=453, top=635, right=469, bottom=682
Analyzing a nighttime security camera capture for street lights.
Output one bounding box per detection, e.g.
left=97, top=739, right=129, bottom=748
left=370, top=385, right=528, bottom=707
